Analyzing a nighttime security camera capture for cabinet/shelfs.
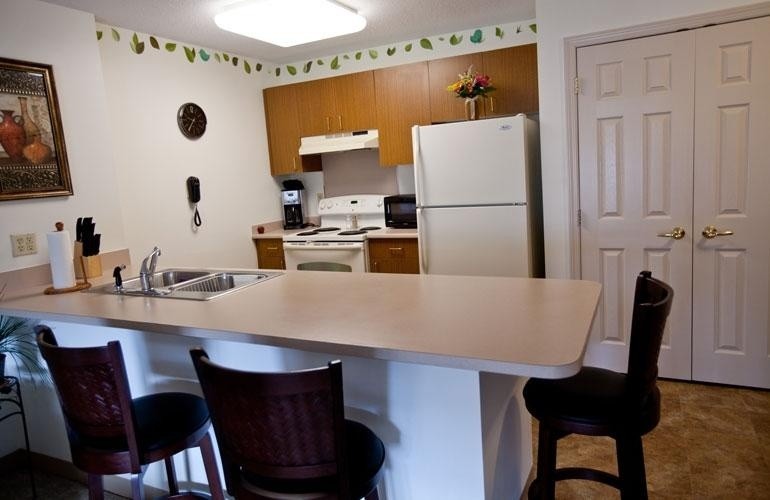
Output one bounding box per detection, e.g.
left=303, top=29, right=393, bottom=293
left=296, top=70, right=375, bottom=136
left=428, top=42, right=538, bottom=123
left=263, top=83, right=322, bottom=175
left=0, top=377, right=37, bottom=500
left=369, top=238, right=419, bottom=274
left=373, top=60, right=431, bottom=167
left=256, top=238, right=286, bottom=269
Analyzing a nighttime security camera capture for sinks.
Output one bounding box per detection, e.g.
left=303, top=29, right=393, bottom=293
left=167, top=270, right=287, bottom=300
left=80, top=268, right=219, bottom=295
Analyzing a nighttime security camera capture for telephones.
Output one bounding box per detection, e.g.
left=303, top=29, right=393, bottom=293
left=187, top=176, right=200, bottom=203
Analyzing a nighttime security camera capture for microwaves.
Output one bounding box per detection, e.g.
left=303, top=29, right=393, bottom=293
left=384, top=195, right=418, bottom=229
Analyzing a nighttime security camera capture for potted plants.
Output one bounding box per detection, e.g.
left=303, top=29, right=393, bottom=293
left=0, top=283, right=53, bottom=388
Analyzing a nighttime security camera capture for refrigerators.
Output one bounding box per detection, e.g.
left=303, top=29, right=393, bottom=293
left=411, top=114, right=541, bottom=276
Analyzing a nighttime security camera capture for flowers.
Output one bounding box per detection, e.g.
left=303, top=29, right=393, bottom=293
left=447, top=64, right=498, bottom=99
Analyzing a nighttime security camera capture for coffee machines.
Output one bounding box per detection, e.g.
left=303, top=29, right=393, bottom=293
left=280, top=181, right=308, bottom=228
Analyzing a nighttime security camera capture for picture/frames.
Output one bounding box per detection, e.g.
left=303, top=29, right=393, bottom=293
left=0, top=56, right=73, bottom=200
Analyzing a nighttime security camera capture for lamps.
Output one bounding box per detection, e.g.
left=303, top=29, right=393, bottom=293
left=213, top=0, right=367, bottom=47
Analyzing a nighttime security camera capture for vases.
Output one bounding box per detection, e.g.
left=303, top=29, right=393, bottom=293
left=464, top=97, right=478, bottom=121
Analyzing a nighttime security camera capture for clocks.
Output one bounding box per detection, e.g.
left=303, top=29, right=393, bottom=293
left=177, top=102, right=207, bottom=138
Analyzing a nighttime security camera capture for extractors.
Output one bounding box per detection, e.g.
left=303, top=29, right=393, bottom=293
left=300, top=129, right=379, bottom=156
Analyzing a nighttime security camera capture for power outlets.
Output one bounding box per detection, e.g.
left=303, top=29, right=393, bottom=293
left=11, top=232, right=37, bottom=257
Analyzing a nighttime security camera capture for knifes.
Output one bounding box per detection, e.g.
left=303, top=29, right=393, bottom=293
left=75, top=216, right=101, bottom=255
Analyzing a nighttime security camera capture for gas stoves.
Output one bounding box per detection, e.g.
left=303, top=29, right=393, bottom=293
left=284, top=223, right=379, bottom=241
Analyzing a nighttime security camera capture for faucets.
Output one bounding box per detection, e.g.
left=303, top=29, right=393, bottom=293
left=140, top=246, right=161, bottom=289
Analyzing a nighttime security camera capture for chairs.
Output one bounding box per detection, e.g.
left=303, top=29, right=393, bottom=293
left=189, top=346, right=386, bottom=500
left=33, top=324, right=224, bottom=500
left=523, top=271, right=674, bottom=499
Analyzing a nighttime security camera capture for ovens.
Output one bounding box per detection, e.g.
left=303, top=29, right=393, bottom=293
left=282, top=242, right=370, bottom=273
